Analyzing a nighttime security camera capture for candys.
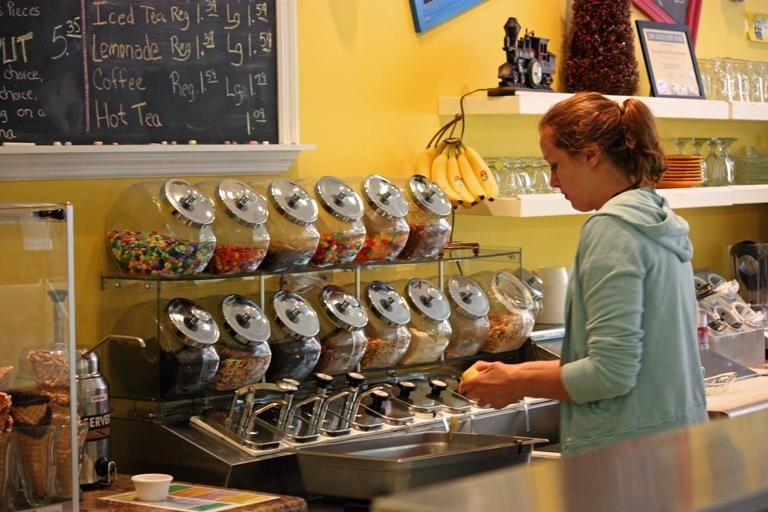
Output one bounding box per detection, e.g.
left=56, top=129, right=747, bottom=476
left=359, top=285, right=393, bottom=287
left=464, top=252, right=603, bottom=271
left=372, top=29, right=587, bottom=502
left=355, top=231, right=407, bottom=262
left=312, top=230, right=364, bottom=264
left=205, top=245, right=267, bottom=273
left=105, top=230, right=214, bottom=277
left=205, top=346, right=270, bottom=391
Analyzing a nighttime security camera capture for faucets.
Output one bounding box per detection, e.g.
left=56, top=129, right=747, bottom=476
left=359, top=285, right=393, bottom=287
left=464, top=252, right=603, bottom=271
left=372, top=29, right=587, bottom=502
left=224, top=383, right=298, bottom=441
left=309, top=373, right=355, bottom=433
left=278, top=378, right=329, bottom=433
left=338, top=372, right=384, bottom=430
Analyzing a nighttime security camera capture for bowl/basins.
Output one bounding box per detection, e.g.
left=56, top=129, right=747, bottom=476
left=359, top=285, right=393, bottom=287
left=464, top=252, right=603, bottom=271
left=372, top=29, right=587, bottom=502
left=131, top=474, right=172, bottom=501
left=536, top=266, right=569, bottom=325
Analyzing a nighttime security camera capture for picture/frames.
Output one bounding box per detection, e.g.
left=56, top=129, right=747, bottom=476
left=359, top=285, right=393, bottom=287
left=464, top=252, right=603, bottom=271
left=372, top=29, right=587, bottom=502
left=636, top=20, right=704, bottom=99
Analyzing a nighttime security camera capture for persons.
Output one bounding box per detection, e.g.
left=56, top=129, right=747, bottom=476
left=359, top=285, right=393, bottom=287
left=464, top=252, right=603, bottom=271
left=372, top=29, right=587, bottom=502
left=458, top=92, right=711, bottom=455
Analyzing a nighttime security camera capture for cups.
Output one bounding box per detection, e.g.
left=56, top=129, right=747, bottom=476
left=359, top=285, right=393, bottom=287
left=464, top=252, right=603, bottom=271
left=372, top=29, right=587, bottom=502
left=706, top=139, right=725, bottom=186
left=482, top=157, right=562, bottom=196
left=692, top=139, right=711, bottom=155
left=670, top=138, right=690, bottom=154
left=696, top=58, right=768, bottom=102
left=717, top=137, right=738, bottom=186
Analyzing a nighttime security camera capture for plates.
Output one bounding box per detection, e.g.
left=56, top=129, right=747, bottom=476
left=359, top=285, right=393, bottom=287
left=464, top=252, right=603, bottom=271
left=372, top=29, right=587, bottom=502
left=653, top=155, right=704, bottom=188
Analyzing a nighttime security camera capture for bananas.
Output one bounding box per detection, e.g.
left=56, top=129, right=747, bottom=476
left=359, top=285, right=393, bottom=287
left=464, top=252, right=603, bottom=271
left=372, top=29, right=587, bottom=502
left=414, top=137, right=497, bottom=209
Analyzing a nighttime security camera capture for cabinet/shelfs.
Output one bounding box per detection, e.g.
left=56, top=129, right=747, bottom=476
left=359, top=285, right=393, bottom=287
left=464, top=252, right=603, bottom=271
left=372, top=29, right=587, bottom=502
left=79, top=475, right=306, bottom=512
left=100, top=243, right=568, bottom=500
